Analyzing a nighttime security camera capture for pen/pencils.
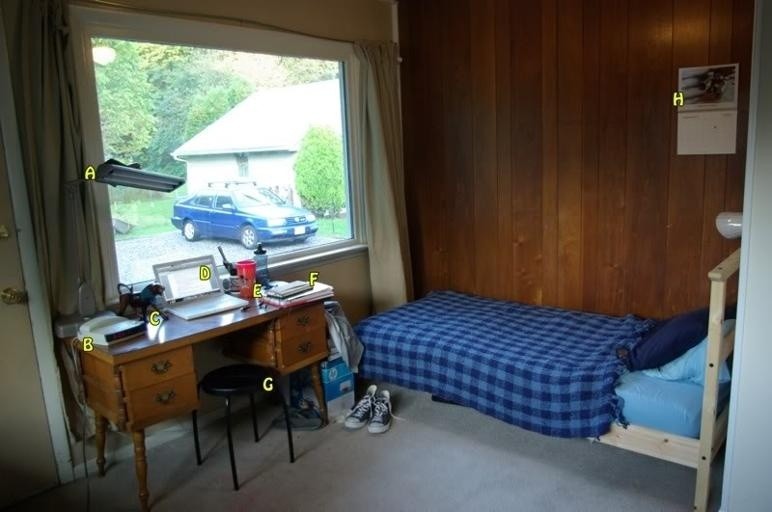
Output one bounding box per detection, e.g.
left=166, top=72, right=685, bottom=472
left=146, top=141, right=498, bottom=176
left=239, top=302, right=267, bottom=311
left=217, top=245, right=237, bottom=275
left=152, top=304, right=168, bottom=320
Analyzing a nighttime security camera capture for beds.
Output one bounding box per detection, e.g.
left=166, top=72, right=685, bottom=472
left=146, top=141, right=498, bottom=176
left=353, top=245, right=740, bottom=511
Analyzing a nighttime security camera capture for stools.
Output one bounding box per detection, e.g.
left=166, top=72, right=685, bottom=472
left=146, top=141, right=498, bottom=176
left=192, top=363, right=296, bottom=491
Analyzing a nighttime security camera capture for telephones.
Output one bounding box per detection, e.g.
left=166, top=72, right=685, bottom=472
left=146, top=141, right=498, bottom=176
left=77, top=316, right=145, bottom=346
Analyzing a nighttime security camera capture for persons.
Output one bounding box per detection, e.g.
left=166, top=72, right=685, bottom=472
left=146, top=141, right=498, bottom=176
left=703, top=72, right=726, bottom=98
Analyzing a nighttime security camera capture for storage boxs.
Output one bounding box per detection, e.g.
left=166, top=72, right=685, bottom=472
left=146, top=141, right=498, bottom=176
left=297, top=357, right=355, bottom=419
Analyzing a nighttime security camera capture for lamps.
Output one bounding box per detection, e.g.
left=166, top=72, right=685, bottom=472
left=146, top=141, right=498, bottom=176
left=60, top=158, right=185, bottom=197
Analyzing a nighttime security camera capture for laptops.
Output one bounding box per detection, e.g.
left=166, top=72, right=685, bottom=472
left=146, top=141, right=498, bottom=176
left=151, top=253, right=250, bottom=321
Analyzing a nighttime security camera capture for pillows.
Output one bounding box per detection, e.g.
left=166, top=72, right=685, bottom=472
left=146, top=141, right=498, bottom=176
left=624, top=301, right=735, bottom=382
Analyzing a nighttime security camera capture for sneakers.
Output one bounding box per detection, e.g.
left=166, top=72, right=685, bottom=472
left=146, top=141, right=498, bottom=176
left=345, top=384, right=394, bottom=434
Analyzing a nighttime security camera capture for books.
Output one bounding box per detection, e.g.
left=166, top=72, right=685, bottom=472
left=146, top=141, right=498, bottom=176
left=261, top=281, right=335, bottom=309
left=266, top=279, right=313, bottom=299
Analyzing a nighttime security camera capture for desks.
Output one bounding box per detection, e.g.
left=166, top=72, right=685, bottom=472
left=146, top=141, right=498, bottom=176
left=64, top=279, right=332, bottom=511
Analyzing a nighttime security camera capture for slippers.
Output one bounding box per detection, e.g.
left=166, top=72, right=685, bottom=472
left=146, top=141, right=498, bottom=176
left=272, top=408, right=321, bottom=431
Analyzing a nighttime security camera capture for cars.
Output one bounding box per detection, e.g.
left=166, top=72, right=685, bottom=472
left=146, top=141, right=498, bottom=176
left=172, top=178, right=318, bottom=250
